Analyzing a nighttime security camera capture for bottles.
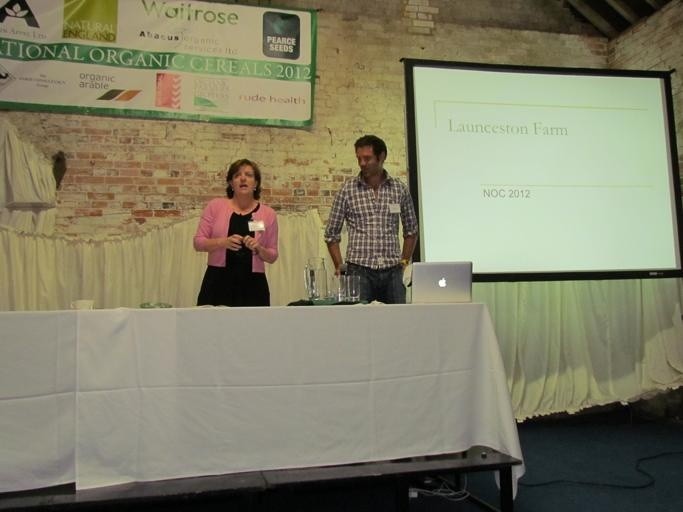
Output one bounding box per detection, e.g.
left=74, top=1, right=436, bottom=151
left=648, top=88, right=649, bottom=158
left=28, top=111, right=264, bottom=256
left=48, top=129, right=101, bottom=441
left=332, top=269, right=359, bottom=304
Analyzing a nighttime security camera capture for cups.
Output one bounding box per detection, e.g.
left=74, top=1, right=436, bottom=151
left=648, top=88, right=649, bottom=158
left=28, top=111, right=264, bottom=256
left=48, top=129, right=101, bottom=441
left=304, top=257, right=327, bottom=301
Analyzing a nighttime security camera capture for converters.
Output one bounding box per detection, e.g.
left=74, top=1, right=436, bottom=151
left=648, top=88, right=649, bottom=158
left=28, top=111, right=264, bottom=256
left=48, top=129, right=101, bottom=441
left=409, top=491, right=419, bottom=499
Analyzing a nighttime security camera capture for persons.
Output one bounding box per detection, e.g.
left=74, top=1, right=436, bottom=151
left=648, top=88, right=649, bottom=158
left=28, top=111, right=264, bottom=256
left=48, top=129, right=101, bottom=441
left=193, top=157, right=278, bottom=306
left=324, top=135, right=417, bottom=304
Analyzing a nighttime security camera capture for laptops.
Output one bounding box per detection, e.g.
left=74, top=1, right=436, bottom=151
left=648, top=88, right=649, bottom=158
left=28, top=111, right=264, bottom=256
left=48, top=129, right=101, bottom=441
left=412, top=260, right=473, bottom=303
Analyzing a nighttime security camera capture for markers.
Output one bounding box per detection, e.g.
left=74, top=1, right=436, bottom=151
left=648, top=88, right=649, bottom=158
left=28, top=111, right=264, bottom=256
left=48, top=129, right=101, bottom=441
left=648, top=272, right=665, bottom=276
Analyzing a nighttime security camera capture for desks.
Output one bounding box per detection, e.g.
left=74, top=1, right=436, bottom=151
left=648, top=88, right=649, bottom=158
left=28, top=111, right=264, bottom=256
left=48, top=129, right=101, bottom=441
left=0, top=303, right=525, bottom=512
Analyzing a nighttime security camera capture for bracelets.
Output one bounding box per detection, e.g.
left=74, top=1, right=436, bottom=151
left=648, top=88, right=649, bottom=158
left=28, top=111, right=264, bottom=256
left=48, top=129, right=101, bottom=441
left=398, top=258, right=410, bottom=266
